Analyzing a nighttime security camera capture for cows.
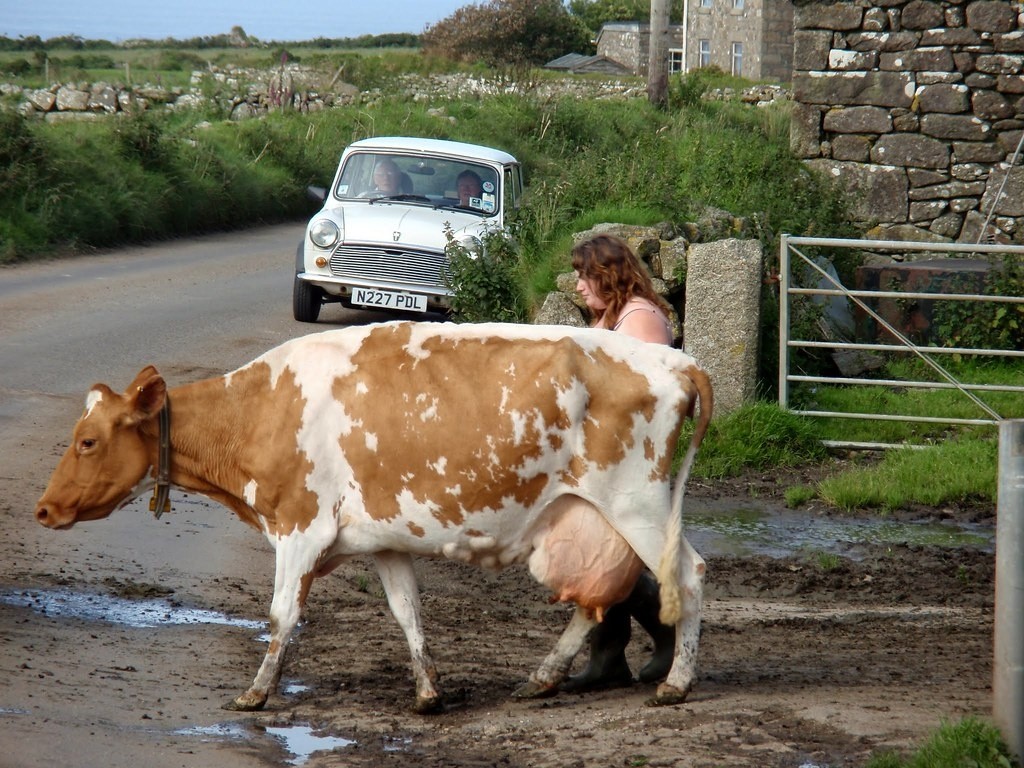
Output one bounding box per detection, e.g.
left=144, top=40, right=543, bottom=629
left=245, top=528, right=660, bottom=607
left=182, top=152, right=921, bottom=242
left=31, top=320, right=717, bottom=714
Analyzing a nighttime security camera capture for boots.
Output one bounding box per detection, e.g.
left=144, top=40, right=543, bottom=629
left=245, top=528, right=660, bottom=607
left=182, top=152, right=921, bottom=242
left=630, top=592, right=677, bottom=683
left=562, top=608, right=632, bottom=692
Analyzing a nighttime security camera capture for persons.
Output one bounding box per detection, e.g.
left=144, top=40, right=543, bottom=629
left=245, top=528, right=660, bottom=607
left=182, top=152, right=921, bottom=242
left=455, top=169, right=484, bottom=208
left=555, top=232, right=671, bottom=693
left=354, top=159, right=404, bottom=202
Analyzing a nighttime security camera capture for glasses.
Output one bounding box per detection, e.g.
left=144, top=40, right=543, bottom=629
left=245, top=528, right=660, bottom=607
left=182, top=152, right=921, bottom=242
left=374, top=170, right=398, bottom=177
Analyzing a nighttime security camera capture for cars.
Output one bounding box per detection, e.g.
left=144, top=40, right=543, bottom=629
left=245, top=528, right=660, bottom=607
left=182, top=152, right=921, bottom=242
left=292, top=135, right=522, bottom=325
